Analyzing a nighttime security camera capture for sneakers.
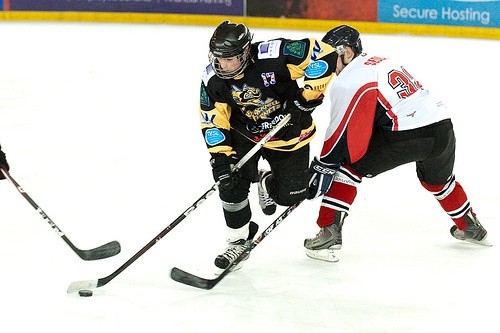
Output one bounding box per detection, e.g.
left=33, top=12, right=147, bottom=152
left=257, top=168, right=277, bottom=215
left=304, top=210, right=348, bottom=261
left=450, top=209, right=493, bottom=246
left=213, top=220, right=259, bottom=276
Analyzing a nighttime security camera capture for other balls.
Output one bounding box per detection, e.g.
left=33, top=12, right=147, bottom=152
left=78, top=290, right=93, bottom=297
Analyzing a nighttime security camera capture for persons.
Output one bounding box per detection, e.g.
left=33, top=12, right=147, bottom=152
left=200, top=20, right=339, bottom=275
left=304, top=24, right=494, bottom=262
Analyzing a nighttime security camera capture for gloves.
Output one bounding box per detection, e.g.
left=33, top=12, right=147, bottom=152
left=210, top=152, right=242, bottom=189
left=303, top=155, right=341, bottom=199
left=288, top=87, right=325, bottom=122
left=0, top=144, right=10, bottom=180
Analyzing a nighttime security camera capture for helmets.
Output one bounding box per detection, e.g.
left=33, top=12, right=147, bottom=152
left=321, top=24, right=363, bottom=54
left=208, top=20, right=253, bottom=78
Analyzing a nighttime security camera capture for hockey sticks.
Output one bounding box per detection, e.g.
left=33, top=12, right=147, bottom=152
left=67, top=112, right=292, bottom=295
left=2, top=167, right=120, bottom=262
left=170, top=196, right=306, bottom=290
